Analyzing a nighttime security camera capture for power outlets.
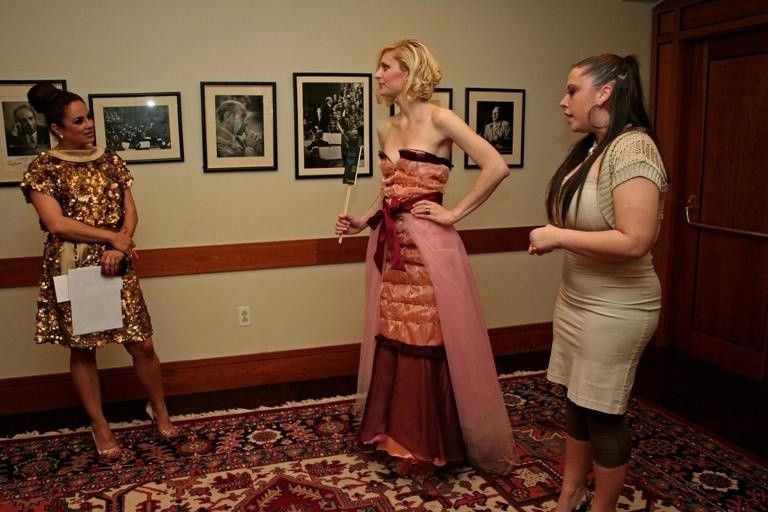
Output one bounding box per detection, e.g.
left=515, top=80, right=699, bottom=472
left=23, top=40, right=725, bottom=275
left=238, top=306, right=250, bottom=327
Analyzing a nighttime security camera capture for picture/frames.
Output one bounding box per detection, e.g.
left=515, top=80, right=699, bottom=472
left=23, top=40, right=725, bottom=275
left=88, top=91, right=185, bottom=165
left=0, top=79, right=67, bottom=187
left=199, top=80, right=279, bottom=174
left=292, top=72, right=374, bottom=180
left=389, top=87, right=453, bottom=172
left=463, top=87, right=524, bottom=170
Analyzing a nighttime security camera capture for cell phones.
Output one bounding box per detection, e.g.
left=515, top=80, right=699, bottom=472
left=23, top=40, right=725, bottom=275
left=343, top=146, right=363, bottom=186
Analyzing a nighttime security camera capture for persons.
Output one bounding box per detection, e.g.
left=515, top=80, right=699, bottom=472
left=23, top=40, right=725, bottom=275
left=524, top=51, right=665, bottom=512
left=19, top=81, right=180, bottom=461
left=335, top=39, right=519, bottom=482
left=482, top=102, right=512, bottom=154
left=10, top=107, right=50, bottom=152
left=216, top=100, right=262, bottom=158
left=303, top=84, right=368, bottom=168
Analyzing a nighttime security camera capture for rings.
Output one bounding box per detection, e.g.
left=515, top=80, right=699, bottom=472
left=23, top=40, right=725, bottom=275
left=423, top=206, right=431, bottom=215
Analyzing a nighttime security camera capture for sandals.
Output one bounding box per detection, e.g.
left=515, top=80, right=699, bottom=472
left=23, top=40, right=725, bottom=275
left=391, top=461, right=436, bottom=479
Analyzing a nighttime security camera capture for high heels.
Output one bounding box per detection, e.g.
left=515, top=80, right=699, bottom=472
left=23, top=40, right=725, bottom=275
left=90, top=428, right=122, bottom=460
left=553, top=488, right=592, bottom=512
left=145, top=400, right=182, bottom=443
